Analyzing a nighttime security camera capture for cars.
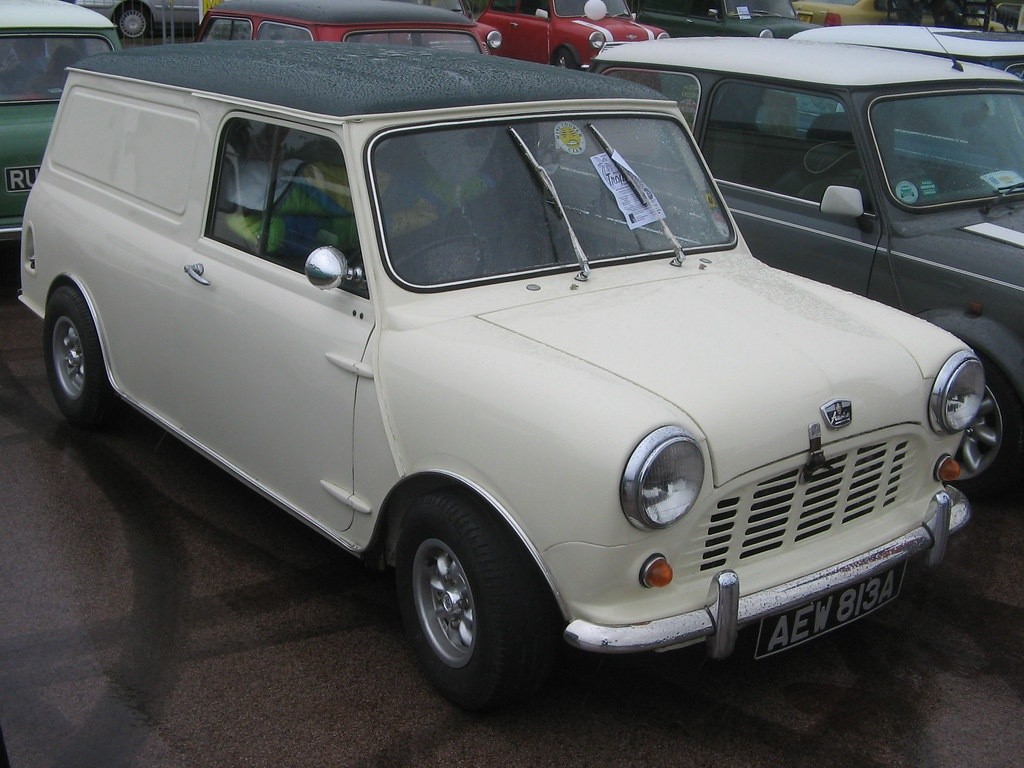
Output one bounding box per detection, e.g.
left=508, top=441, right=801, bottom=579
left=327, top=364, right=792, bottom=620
left=191, top=0, right=507, bottom=55
left=789, top=23, right=1024, bottom=94
left=474, top=1, right=672, bottom=72
left=16, top=42, right=1005, bottom=721
left=74, top=0, right=199, bottom=42
left=0, top=0, right=125, bottom=247
left=791, top=0, right=1024, bottom=37
left=625, top=0, right=829, bottom=41
left=554, top=37, right=1024, bottom=514
